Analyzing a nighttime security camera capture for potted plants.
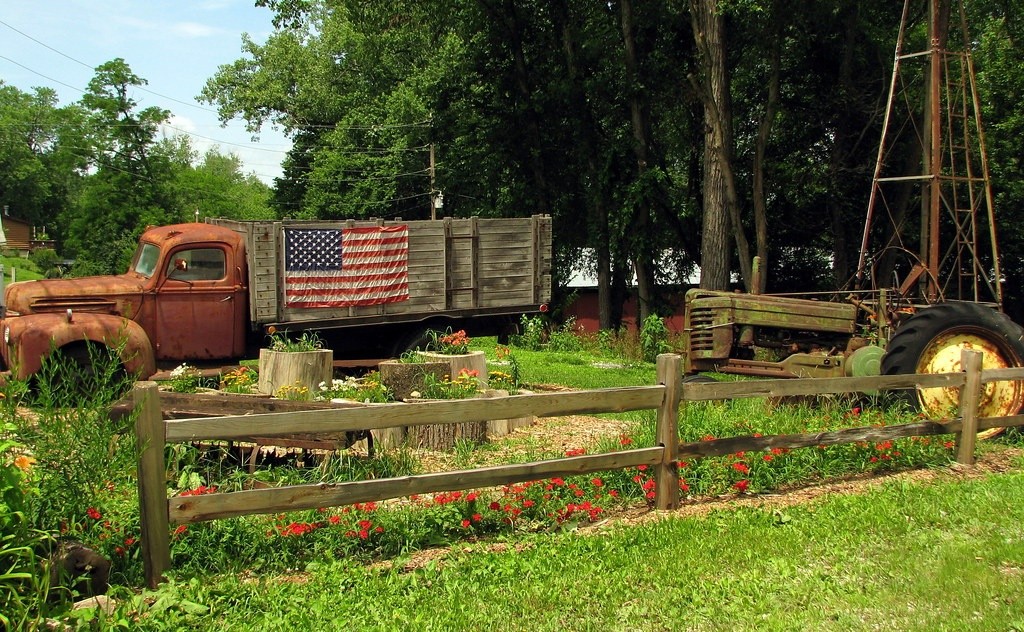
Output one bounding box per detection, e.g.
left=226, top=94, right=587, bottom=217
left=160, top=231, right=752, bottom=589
left=353, top=349, right=534, bottom=451
left=257, top=331, right=333, bottom=397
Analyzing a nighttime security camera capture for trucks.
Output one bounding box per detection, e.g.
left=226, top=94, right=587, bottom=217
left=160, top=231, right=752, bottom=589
left=0, top=215, right=554, bottom=406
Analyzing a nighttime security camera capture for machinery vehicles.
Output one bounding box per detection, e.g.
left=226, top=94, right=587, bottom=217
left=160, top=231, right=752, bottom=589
left=683, top=256, right=1024, bottom=444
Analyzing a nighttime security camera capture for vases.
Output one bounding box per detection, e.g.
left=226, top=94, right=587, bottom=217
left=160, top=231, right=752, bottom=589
left=416, top=351, right=489, bottom=389
left=480, top=389, right=510, bottom=438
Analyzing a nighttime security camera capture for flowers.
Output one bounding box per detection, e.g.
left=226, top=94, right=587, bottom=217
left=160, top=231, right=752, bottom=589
left=425, top=328, right=469, bottom=354
left=457, top=369, right=479, bottom=390
